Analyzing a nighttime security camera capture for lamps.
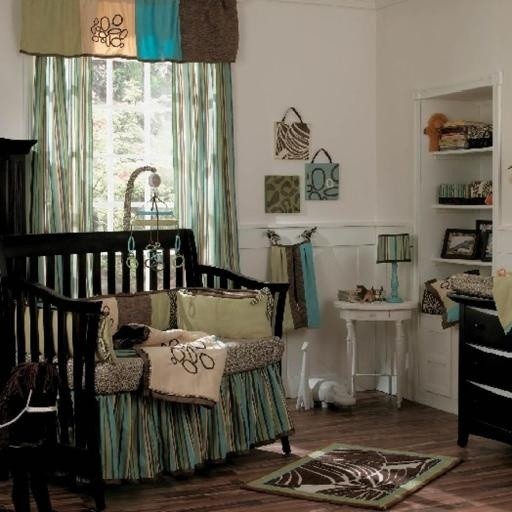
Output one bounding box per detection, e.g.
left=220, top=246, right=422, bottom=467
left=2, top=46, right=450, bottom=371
left=377, top=232, right=412, bottom=304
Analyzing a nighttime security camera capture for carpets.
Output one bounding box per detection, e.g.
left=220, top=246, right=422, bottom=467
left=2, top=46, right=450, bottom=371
left=239, top=441, right=463, bottom=511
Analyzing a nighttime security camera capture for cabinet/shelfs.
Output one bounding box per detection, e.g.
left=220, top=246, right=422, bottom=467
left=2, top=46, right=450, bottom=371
left=446, top=290, right=512, bottom=448
left=413, top=71, right=499, bottom=416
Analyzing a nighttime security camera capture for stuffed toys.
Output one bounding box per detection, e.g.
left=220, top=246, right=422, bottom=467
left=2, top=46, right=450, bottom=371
left=424, top=113, right=448, bottom=151
left=356, top=285, right=375, bottom=304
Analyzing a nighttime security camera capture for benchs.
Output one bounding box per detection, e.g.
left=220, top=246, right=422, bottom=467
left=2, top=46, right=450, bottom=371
left=0, top=229, right=295, bottom=511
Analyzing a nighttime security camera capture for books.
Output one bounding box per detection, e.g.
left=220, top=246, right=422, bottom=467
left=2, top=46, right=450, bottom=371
left=438, top=120, right=469, bottom=150
left=440, top=181, right=492, bottom=205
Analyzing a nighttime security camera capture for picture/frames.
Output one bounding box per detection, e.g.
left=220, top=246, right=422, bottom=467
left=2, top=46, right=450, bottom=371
left=440, top=229, right=482, bottom=259
left=481, top=230, right=493, bottom=262
left=476, top=219, right=492, bottom=242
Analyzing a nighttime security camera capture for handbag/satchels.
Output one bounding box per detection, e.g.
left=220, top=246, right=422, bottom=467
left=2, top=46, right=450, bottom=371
left=273, top=107, right=310, bottom=161
left=304, top=148, right=339, bottom=200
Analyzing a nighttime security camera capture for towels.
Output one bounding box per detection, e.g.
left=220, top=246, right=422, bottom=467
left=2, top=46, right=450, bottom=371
left=264, top=240, right=320, bottom=332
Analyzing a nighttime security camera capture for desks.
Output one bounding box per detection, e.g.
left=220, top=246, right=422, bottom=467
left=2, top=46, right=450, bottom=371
left=333, top=297, right=419, bottom=410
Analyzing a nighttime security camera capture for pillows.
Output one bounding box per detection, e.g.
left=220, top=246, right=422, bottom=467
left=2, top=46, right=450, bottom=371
left=14, top=304, right=118, bottom=368
left=87, top=288, right=177, bottom=339
left=176, top=286, right=272, bottom=339
